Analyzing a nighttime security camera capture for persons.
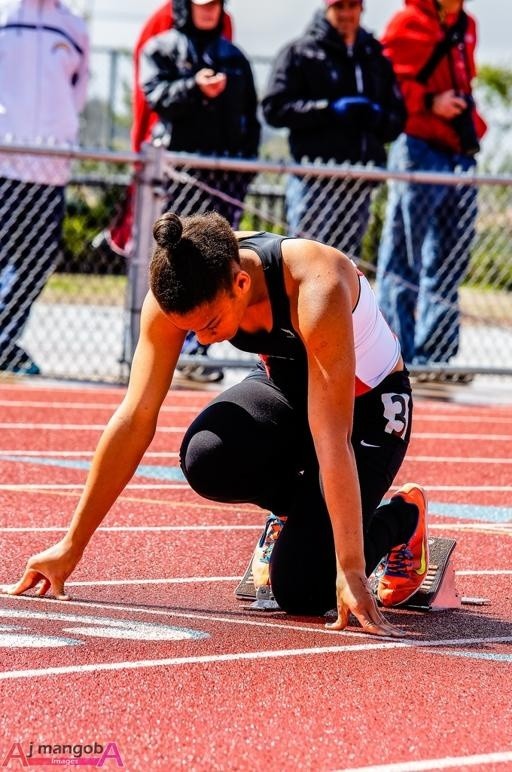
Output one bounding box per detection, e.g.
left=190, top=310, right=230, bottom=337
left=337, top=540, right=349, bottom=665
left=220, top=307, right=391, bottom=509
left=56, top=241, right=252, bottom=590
left=1, top=0, right=94, bottom=380
left=120, top=0, right=263, bottom=382
left=262, top=0, right=410, bottom=262
left=382, top=0, right=489, bottom=384
left=0, top=210, right=431, bottom=640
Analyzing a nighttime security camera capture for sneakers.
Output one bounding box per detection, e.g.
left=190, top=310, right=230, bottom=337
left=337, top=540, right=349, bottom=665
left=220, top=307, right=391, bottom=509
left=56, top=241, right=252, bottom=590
left=377, top=483, right=430, bottom=607
left=177, top=349, right=224, bottom=383
left=252, top=516, right=287, bottom=591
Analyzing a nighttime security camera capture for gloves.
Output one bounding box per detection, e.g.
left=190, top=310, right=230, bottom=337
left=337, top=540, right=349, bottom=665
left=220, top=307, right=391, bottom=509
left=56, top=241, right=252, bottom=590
left=335, top=97, right=381, bottom=121
